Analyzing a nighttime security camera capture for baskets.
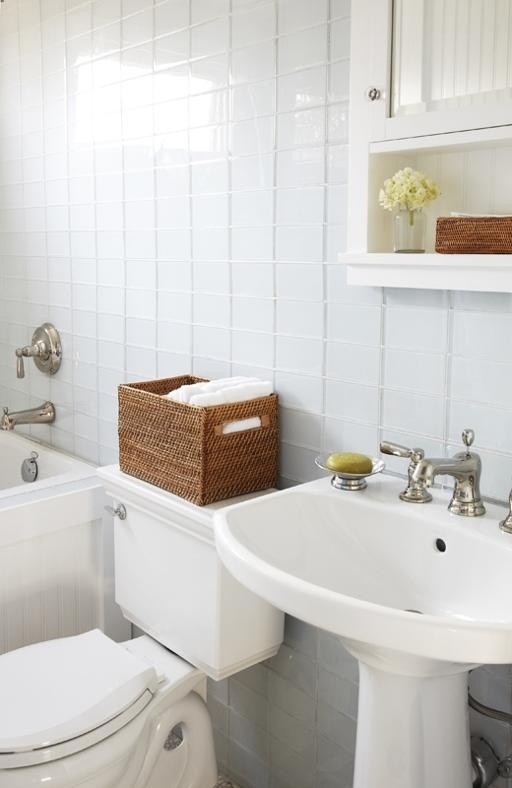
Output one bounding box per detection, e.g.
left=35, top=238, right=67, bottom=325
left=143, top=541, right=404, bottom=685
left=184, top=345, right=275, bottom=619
left=435, top=217, right=511, bottom=253
left=119, top=375, right=279, bottom=506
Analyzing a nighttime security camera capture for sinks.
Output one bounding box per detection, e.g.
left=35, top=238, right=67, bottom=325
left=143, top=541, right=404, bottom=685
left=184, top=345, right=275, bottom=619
left=214, top=476, right=512, bottom=665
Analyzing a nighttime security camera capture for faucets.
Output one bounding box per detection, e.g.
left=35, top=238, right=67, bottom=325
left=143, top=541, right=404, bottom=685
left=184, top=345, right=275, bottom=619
left=399, top=429, right=487, bottom=517
left=0, top=401, right=55, bottom=431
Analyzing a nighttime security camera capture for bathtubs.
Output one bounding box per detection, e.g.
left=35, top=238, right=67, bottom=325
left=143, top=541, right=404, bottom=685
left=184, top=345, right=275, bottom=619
left=0, top=430, right=131, bottom=652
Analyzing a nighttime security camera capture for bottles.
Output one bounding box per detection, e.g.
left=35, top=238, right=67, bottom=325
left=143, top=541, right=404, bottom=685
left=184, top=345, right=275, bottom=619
left=394, top=208, right=427, bottom=253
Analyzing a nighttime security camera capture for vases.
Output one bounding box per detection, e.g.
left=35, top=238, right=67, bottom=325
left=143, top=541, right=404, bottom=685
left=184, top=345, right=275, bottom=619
left=391, top=207, right=429, bottom=254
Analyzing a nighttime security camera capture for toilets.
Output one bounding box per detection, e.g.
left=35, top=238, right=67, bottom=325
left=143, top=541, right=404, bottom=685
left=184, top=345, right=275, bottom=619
left=0, top=465, right=286, bottom=788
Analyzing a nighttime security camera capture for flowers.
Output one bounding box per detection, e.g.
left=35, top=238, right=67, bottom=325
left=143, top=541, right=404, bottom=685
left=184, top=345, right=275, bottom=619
left=376, top=166, right=439, bottom=228
